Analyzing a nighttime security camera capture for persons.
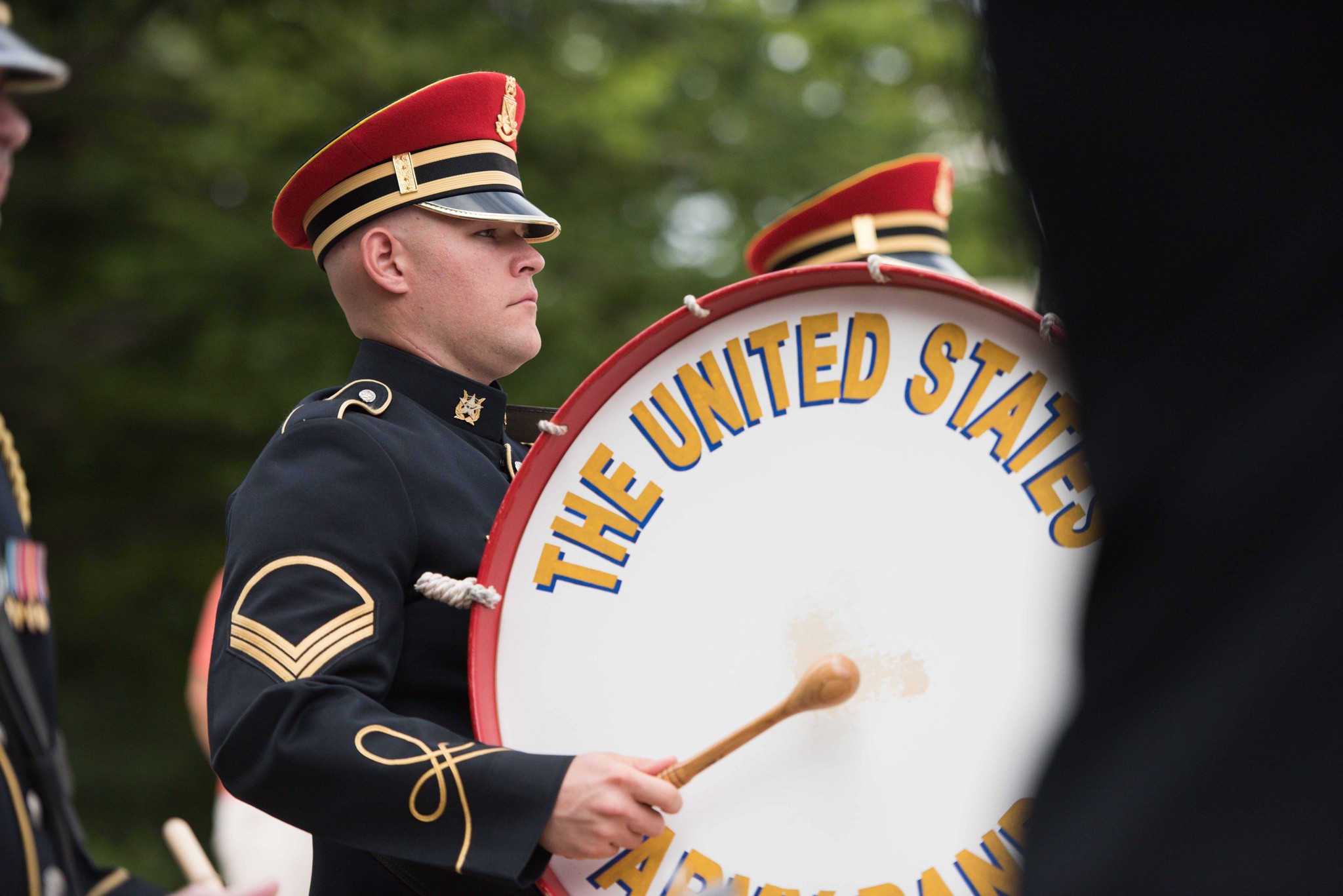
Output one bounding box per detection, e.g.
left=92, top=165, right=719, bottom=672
left=980, top=0, right=1343, bottom=896
left=745, top=153, right=979, bottom=287
left=0, top=0, right=278, bottom=895
left=206, top=71, right=684, bottom=896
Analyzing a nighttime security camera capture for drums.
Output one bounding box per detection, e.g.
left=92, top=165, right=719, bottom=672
left=466, top=261, right=1107, bottom=896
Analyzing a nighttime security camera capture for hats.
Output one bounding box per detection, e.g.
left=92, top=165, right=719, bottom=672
left=0, top=2, right=67, bottom=94
left=272, top=72, right=561, bottom=271
left=741, top=151, right=978, bottom=288
